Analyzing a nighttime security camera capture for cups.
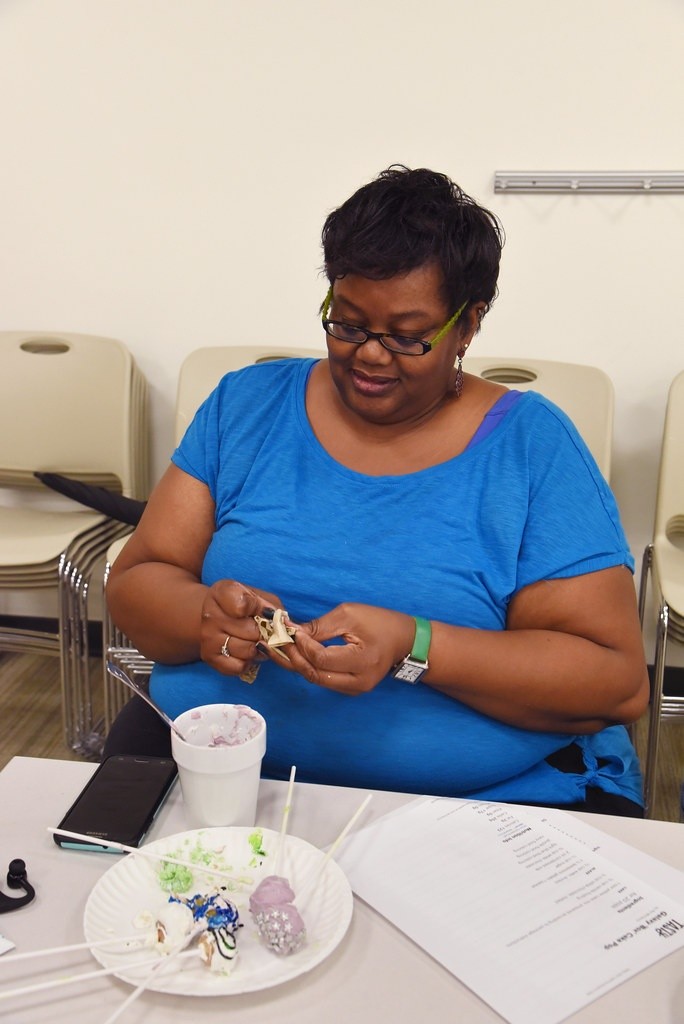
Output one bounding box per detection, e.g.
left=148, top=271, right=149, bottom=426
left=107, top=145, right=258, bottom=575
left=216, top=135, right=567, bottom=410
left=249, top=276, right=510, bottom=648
left=170, top=705, right=266, bottom=827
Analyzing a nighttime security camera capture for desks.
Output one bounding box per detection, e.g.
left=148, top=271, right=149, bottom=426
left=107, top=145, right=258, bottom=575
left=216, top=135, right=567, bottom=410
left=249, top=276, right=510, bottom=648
left=1, top=755, right=684, bottom=1023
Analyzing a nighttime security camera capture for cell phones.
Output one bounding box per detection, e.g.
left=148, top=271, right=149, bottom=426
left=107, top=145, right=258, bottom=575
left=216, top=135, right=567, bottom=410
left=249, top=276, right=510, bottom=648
left=52, top=753, right=179, bottom=853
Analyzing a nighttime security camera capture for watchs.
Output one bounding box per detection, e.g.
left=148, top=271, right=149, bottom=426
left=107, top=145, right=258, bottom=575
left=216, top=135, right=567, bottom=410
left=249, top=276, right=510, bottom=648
left=391, top=616, right=431, bottom=685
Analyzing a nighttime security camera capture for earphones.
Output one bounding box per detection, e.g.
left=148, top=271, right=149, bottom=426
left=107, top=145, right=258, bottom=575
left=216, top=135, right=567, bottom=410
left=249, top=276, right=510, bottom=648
left=7, top=859, right=27, bottom=889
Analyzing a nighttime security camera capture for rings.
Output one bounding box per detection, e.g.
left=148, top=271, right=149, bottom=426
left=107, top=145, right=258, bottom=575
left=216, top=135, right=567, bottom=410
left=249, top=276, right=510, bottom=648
left=221, top=636, right=231, bottom=657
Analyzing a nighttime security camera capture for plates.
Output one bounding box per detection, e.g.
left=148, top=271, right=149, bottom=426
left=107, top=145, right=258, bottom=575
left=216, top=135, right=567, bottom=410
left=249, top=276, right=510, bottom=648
left=83, top=828, right=353, bottom=998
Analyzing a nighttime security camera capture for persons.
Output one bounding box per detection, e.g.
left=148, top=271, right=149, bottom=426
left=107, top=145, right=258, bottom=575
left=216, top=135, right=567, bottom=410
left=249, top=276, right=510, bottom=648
left=103, top=164, right=650, bottom=819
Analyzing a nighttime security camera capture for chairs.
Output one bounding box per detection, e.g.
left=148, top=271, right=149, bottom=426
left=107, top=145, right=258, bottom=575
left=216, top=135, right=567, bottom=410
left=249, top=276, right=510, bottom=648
left=0, top=328, right=326, bottom=760
left=454, top=356, right=684, bottom=822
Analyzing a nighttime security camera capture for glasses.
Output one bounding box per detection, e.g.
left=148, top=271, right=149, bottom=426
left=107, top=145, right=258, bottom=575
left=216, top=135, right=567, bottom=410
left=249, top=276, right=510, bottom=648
left=321, top=284, right=471, bottom=357
